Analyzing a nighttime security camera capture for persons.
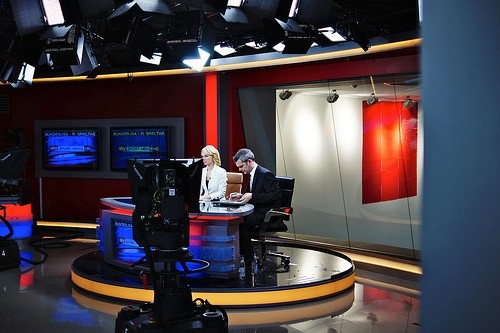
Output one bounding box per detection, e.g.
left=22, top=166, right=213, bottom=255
left=229, top=149, right=281, bottom=277
left=199, top=145, right=227, bottom=200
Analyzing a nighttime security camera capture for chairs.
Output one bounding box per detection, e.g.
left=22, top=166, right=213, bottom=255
left=225, top=172, right=295, bottom=276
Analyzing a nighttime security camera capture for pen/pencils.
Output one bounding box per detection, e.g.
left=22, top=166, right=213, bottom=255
left=232, top=194, right=243, bottom=196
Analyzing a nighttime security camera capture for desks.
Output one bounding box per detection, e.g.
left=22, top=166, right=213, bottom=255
left=95, top=196, right=255, bottom=280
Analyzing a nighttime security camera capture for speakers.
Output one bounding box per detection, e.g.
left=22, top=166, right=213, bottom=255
left=0, top=239, right=20, bottom=272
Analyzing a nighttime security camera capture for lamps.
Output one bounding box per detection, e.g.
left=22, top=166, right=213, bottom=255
left=366, top=92, right=378, bottom=105
left=403, top=95, right=412, bottom=108
left=279, top=91, right=293, bottom=100
left=326, top=89, right=339, bottom=104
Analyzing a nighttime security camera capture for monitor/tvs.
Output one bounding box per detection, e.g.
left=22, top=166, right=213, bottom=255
left=40, top=127, right=101, bottom=172
left=109, top=126, right=171, bottom=172
left=125, top=157, right=204, bottom=214
